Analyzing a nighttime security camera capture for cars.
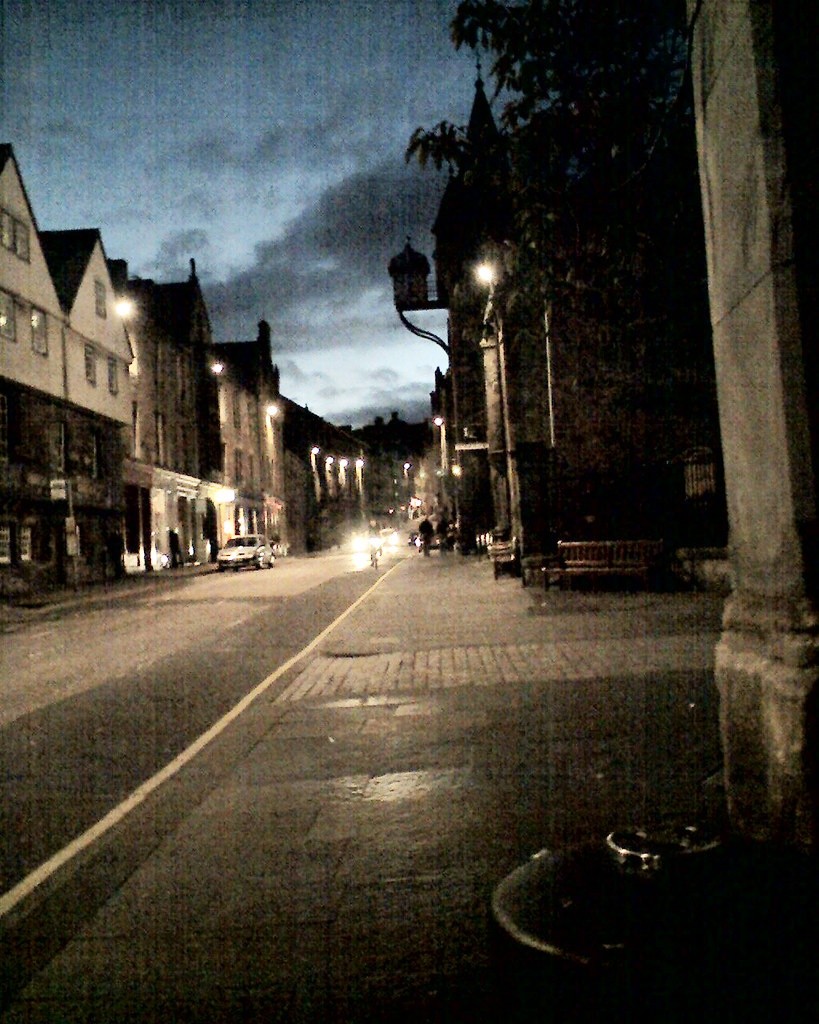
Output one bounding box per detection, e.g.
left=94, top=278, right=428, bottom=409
left=217, top=534, right=274, bottom=571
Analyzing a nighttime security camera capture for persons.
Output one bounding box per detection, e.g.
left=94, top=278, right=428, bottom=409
left=436, top=512, right=459, bottom=553
left=408, top=538, right=417, bottom=548
left=305, top=532, right=316, bottom=553
left=368, top=519, right=381, bottom=535
left=418, top=515, right=435, bottom=557
left=169, top=529, right=180, bottom=568
left=538, top=520, right=565, bottom=558
left=383, top=503, right=421, bottom=523
left=98, top=546, right=110, bottom=579
left=368, top=540, right=385, bottom=567
left=321, top=516, right=329, bottom=527
left=81, top=534, right=97, bottom=564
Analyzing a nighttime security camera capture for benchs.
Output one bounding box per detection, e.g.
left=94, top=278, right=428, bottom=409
left=541, top=538, right=676, bottom=592
left=487, top=534, right=522, bottom=581
